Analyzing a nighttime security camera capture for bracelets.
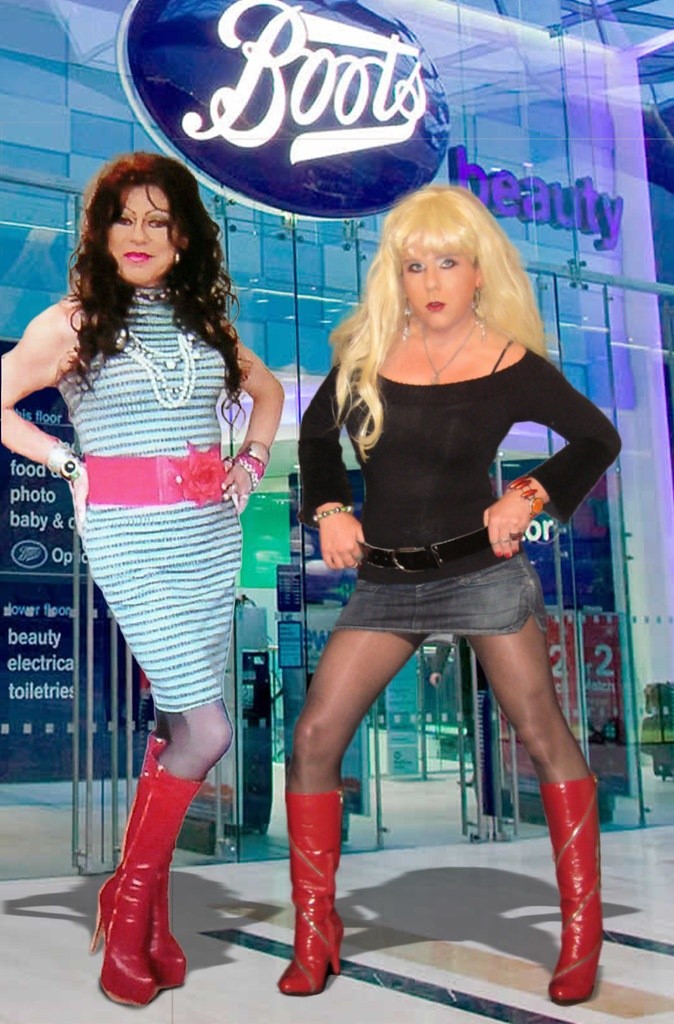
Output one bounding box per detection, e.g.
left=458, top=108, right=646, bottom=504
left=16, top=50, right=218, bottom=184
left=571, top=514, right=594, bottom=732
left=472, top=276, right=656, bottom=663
left=507, top=476, right=542, bottom=520
left=236, top=442, right=271, bottom=491
left=46, top=438, right=81, bottom=483
left=313, top=506, right=353, bottom=521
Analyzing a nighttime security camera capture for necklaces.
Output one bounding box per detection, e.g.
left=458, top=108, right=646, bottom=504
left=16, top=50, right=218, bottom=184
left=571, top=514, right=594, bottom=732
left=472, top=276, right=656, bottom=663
left=120, top=330, right=196, bottom=408
left=422, top=320, right=476, bottom=385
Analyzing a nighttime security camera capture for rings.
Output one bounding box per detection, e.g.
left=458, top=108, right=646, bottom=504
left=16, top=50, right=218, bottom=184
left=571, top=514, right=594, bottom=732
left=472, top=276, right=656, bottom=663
left=232, top=481, right=239, bottom=491
left=490, top=531, right=523, bottom=545
left=350, top=560, right=358, bottom=569
left=240, top=495, right=249, bottom=500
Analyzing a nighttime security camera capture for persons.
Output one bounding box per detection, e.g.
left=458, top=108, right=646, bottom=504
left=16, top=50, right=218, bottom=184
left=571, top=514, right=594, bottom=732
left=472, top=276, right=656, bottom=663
left=3, top=153, right=288, bottom=1006
left=280, top=187, right=623, bottom=1005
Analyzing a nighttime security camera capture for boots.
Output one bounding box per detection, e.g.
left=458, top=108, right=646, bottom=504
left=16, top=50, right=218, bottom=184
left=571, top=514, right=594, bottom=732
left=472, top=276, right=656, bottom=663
left=277, top=784, right=343, bottom=995
left=89, top=730, right=205, bottom=1009
left=539, top=774, right=603, bottom=1004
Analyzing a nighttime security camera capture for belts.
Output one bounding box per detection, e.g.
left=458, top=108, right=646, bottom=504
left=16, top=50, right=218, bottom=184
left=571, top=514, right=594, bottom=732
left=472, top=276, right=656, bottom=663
left=359, top=526, right=488, bottom=573
